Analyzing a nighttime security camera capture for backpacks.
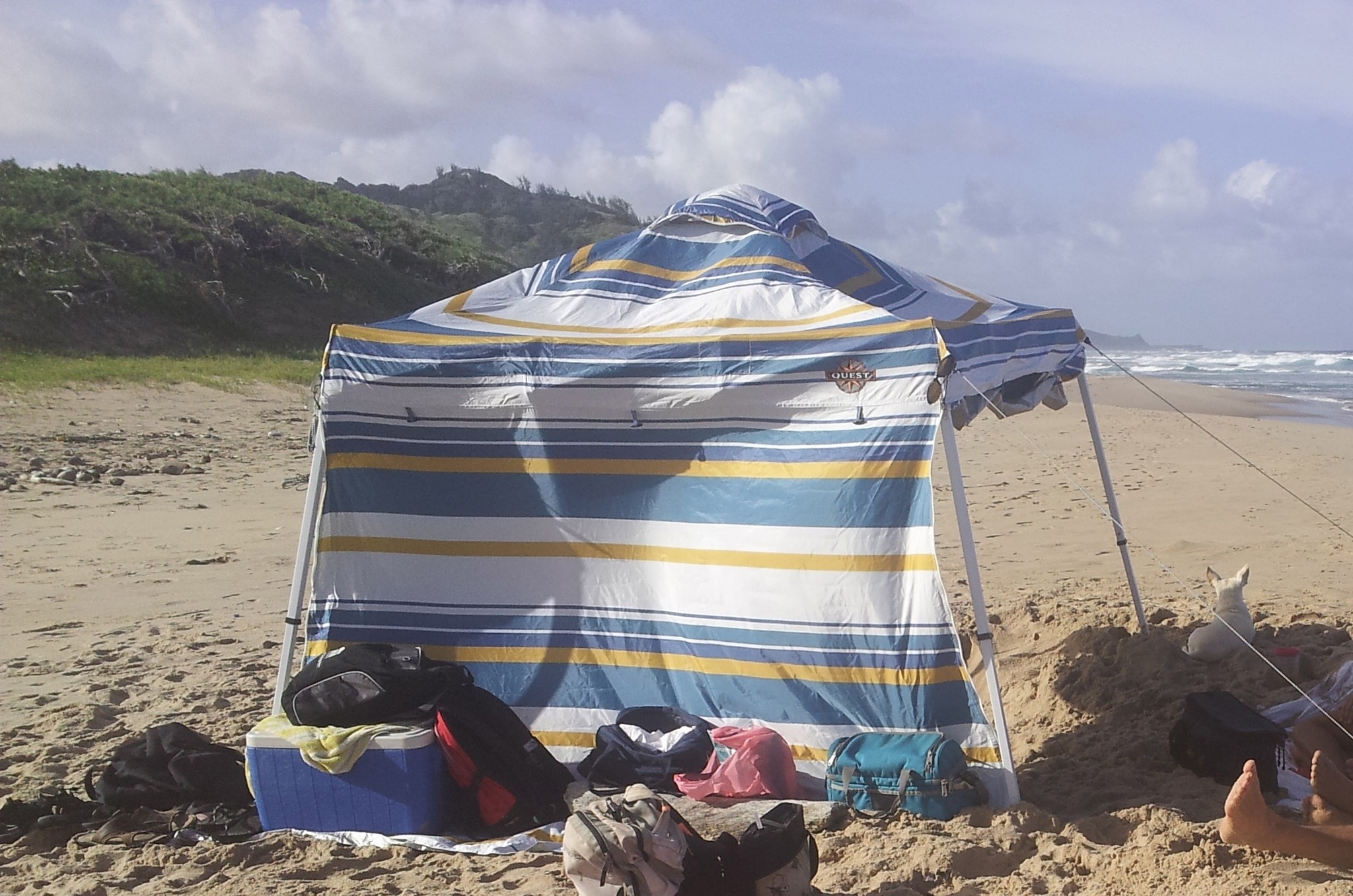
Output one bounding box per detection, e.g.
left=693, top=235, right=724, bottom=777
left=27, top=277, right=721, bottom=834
left=431, top=684, right=575, bottom=836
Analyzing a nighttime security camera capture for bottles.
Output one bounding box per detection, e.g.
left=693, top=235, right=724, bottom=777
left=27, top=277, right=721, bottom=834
left=1298, top=766, right=1311, bottom=778
left=1277, top=647, right=1301, bottom=680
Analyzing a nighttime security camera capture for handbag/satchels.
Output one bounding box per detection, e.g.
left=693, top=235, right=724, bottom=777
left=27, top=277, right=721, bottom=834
left=563, top=783, right=688, bottom=896
left=664, top=799, right=820, bottom=896
left=579, top=706, right=721, bottom=796
left=673, top=725, right=799, bottom=799
left=84, top=722, right=256, bottom=809
left=1170, top=691, right=1288, bottom=793
left=825, top=731, right=989, bottom=821
left=281, top=641, right=465, bottom=728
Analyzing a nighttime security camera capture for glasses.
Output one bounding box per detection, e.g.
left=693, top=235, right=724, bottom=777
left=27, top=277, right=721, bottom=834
left=926, top=354, right=956, bottom=404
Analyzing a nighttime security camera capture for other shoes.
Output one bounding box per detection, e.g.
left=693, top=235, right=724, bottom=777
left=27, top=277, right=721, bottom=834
left=0, top=783, right=85, bottom=846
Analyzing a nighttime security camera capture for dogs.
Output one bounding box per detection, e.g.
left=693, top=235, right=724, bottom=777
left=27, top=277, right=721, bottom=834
left=1180, top=562, right=1258, bottom=663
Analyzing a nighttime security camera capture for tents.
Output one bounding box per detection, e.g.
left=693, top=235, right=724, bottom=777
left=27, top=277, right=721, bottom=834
left=269, top=183, right=1148, bottom=811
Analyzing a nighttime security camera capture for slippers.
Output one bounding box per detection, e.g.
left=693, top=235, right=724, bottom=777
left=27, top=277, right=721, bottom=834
left=81, top=807, right=197, bottom=833
left=185, top=807, right=263, bottom=844
left=77, top=816, right=171, bottom=849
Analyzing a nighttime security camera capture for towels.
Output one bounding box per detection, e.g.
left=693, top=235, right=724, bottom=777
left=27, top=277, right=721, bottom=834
left=243, top=710, right=421, bottom=801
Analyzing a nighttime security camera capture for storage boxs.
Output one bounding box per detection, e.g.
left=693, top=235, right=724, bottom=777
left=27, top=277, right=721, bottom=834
left=245, top=711, right=452, bottom=837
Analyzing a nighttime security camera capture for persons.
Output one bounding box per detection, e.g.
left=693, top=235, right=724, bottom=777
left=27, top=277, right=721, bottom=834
left=1221, top=692, right=1353, bottom=867
